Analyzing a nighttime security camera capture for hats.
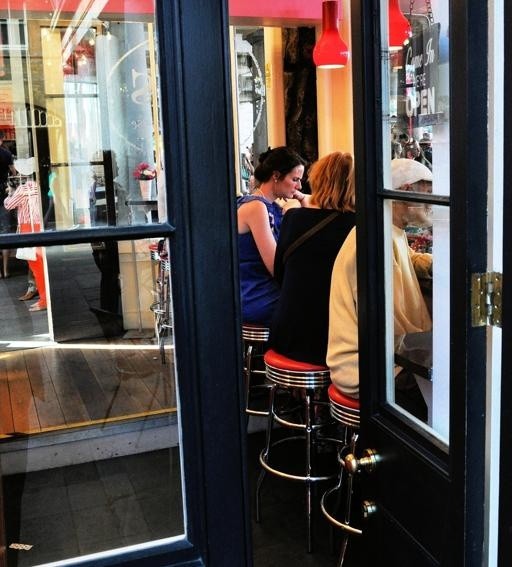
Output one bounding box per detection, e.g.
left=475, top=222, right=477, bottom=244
left=390, top=158, right=432, bottom=189
left=13, top=157, right=36, bottom=176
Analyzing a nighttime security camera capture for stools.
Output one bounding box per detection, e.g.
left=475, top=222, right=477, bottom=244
left=242, top=326, right=363, bottom=567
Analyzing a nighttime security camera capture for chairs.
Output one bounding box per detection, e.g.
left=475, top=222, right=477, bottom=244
left=149, top=242, right=173, bottom=365
left=89, top=308, right=173, bottom=447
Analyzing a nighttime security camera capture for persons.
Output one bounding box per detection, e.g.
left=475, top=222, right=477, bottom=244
left=0, top=128, right=57, bottom=314
left=321, top=155, right=438, bottom=404
left=391, top=130, right=432, bottom=170
left=233, top=145, right=310, bottom=319
left=268, top=149, right=355, bottom=375
left=87, top=149, right=135, bottom=313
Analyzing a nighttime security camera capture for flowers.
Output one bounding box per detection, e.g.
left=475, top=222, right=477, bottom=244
left=132, top=162, right=156, bottom=180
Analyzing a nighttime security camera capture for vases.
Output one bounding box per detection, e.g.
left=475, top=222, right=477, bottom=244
left=139, top=180, right=152, bottom=201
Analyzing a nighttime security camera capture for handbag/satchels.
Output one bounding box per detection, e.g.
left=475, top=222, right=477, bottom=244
left=15, top=246, right=37, bottom=261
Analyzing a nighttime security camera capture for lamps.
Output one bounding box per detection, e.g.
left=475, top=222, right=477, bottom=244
left=389, top=0, right=412, bottom=51
left=312, top=1, right=348, bottom=69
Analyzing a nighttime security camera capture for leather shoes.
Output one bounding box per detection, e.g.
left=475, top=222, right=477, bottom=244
left=18, top=290, right=34, bottom=301
left=29, top=302, right=38, bottom=308
left=28, top=305, right=42, bottom=312
